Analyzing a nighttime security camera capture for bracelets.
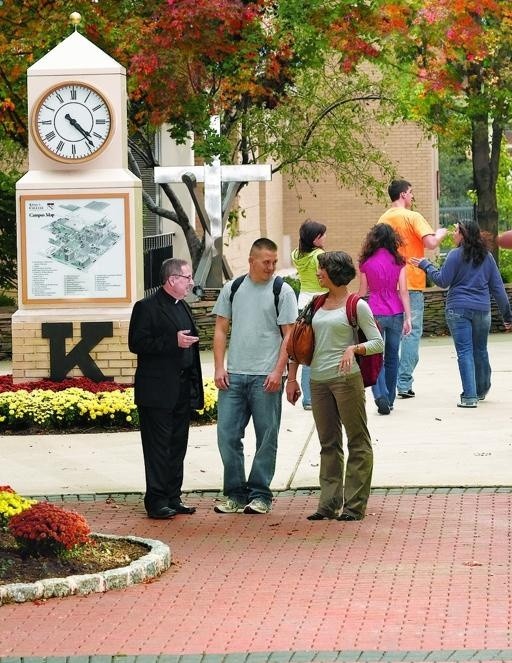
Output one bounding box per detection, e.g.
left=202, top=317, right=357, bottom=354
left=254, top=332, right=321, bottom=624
left=288, top=379, right=296, bottom=382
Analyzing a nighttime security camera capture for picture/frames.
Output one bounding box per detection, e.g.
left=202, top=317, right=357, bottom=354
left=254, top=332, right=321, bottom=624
left=19, top=193, right=132, bottom=305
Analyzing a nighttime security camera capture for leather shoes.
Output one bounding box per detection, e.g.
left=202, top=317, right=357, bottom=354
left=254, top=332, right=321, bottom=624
left=336, top=513, right=356, bottom=521
left=169, top=502, right=196, bottom=514
left=147, top=505, right=178, bottom=519
left=307, top=513, right=325, bottom=521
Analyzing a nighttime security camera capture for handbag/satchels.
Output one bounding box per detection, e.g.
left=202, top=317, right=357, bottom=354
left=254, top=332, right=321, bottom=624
left=286, top=294, right=319, bottom=366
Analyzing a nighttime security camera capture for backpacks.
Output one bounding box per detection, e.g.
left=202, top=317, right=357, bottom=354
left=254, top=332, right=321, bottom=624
left=313, top=294, right=383, bottom=388
left=231, top=275, right=284, bottom=316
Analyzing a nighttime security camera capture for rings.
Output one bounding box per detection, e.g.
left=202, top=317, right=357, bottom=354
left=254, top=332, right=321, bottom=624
left=297, top=390, right=301, bottom=393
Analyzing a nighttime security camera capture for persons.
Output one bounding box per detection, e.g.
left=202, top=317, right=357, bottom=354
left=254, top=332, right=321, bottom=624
left=376, top=181, right=449, bottom=398
left=357, top=223, right=411, bottom=414
left=286, top=251, right=386, bottom=523
left=292, top=220, right=328, bottom=410
left=128, top=258, right=204, bottom=519
left=210, top=237, right=298, bottom=514
left=409, top=218, right=512, bottom=408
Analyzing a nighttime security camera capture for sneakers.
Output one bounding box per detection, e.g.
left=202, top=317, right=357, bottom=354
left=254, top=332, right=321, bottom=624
left=457, top=397, right=478, bottom=408
left=214, top=498, right=248, bottom=514
left=397, top=389, right=416, bottom=397
left=374, top=397, right=390, bottom=416
left=243, top=497, right=271, bottom=514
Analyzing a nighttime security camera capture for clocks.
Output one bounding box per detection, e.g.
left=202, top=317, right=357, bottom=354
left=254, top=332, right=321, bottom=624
left=29, top=79, right=114, bottom=165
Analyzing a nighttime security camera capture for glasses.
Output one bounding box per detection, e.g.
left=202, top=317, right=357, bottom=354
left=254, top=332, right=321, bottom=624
left=171, top=273, right=192, bottom=281
